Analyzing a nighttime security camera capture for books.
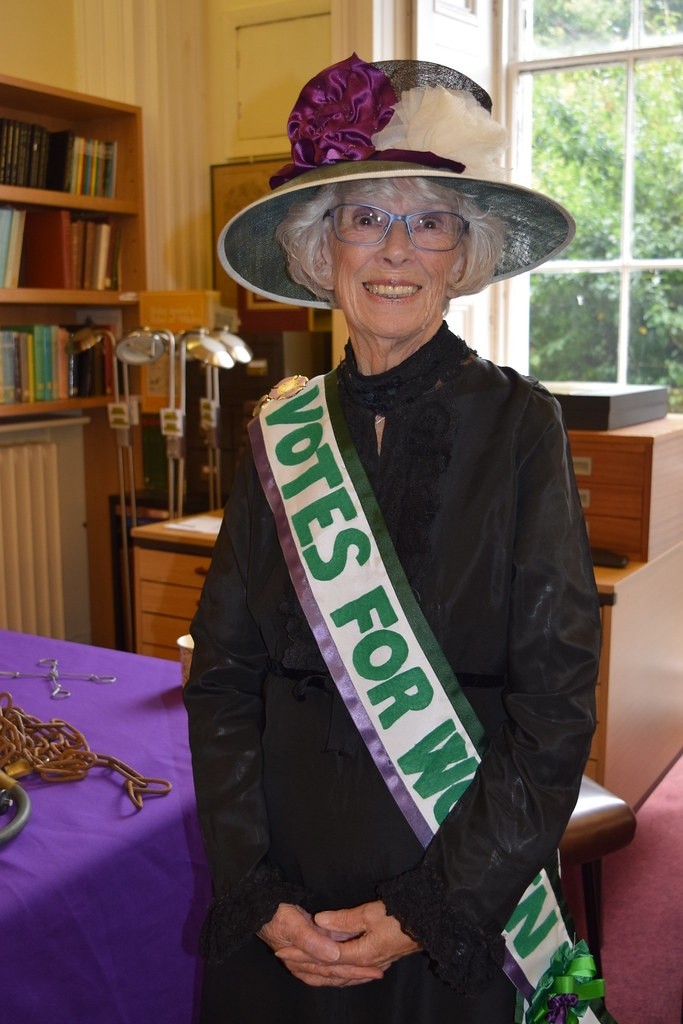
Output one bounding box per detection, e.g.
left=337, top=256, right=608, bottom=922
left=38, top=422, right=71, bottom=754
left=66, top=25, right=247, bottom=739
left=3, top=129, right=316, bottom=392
left=0, top=120, right=122, bottom=403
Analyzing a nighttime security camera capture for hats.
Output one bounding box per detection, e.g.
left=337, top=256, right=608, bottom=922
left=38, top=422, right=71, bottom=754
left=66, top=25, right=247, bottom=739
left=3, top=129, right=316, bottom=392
left=216, top=52, right=576, bottom=310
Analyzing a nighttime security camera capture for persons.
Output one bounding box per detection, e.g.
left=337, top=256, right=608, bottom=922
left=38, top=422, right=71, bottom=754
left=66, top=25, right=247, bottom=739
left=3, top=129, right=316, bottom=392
left=183, top=54, right=602, bottom=1024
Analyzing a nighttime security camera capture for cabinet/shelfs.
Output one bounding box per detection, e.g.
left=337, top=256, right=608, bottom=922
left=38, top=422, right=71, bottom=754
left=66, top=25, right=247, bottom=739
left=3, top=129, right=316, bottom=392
left=131, top=418, right=683, bottom=813
left=0, top=72, right=146, bottom=648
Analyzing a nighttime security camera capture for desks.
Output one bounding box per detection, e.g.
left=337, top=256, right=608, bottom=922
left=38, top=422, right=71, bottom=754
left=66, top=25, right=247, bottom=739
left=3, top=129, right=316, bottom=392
left=0, top=630, right=212, bottom=1024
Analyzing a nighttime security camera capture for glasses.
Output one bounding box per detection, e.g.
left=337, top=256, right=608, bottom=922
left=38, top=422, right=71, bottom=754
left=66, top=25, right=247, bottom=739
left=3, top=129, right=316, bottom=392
left=322, top=203, right=470, bottom=251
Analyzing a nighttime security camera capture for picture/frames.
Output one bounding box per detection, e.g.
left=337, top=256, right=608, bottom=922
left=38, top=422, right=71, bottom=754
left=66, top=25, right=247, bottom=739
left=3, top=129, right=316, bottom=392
left=210, top=157, right=309, bottom=331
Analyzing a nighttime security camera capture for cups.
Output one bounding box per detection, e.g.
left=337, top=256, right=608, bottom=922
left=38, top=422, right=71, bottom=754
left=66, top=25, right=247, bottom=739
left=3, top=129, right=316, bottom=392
left=177, top=634, right=194, bottom=687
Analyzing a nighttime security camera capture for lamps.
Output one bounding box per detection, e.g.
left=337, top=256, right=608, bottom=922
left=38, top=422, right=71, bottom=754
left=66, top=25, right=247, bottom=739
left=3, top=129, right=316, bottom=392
left=65, top=326, right=253, bottom=654
